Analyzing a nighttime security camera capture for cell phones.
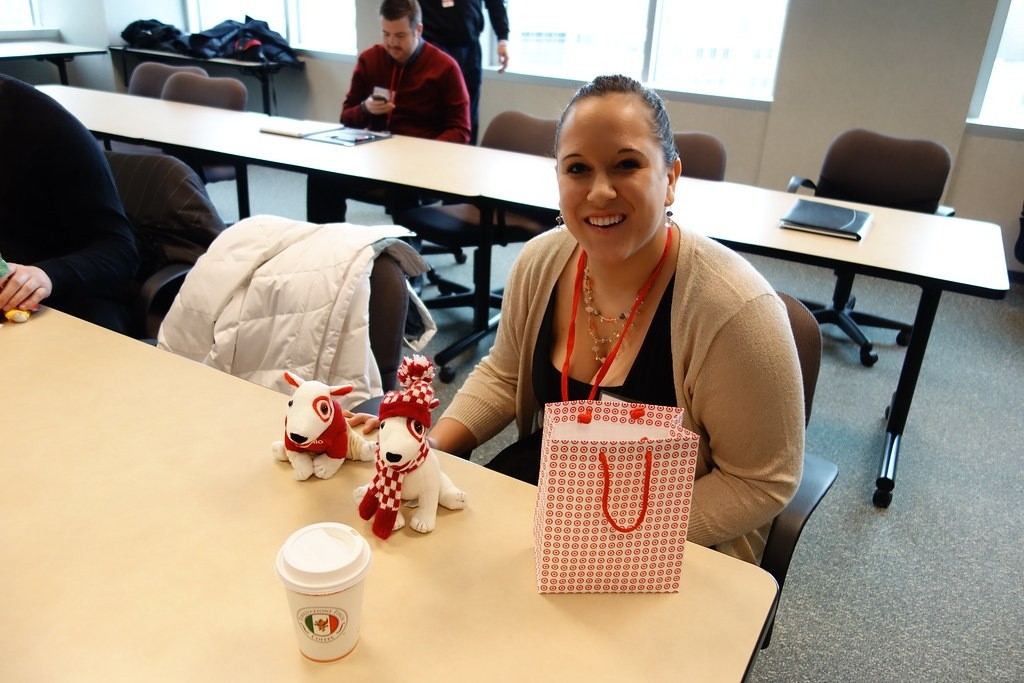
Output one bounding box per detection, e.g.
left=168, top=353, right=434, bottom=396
left=371, top=95, right=389, bottom=104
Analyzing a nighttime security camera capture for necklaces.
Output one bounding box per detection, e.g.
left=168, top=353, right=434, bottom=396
left=583, top=257, right=656, bottom=365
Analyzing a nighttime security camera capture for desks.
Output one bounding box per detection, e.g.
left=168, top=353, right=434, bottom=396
left=0, top=37, right=107, bottom=85
left=1, top=288, right=779, bottom=683
left=110, top=44, right=282, bottom=115
left=31, top=78, right=1010, bottom=510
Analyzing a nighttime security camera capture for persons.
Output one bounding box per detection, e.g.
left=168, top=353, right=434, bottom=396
left=0, top=71, right=138, bottom=336
left=339, top=0, right=510, bottom=295
left=342, top=73, right=804, bottom=565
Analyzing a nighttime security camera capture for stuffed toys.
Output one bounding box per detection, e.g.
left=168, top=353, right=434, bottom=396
left=269, top=353, right=466, bottom=539
left=0, top=255, right=42, bottom=327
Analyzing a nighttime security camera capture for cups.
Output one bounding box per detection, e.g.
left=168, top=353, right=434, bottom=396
left=275, top=521, right=371, bottom=663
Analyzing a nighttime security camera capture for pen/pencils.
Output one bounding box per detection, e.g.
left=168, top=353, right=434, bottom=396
left=356, top=136, right=375, bottom=140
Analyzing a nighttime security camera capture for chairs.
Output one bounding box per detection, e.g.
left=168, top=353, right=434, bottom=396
left=775, top=127, right=950, bottom=366
left=484, top=289, right=842, bottom=646
left=391, top=111, right=727, bottom=379
left=144, top=217, right=410, bottom=421
left=127, top=61, right=208, bottom=104
left=159, top=72, right=254, bottom=220
